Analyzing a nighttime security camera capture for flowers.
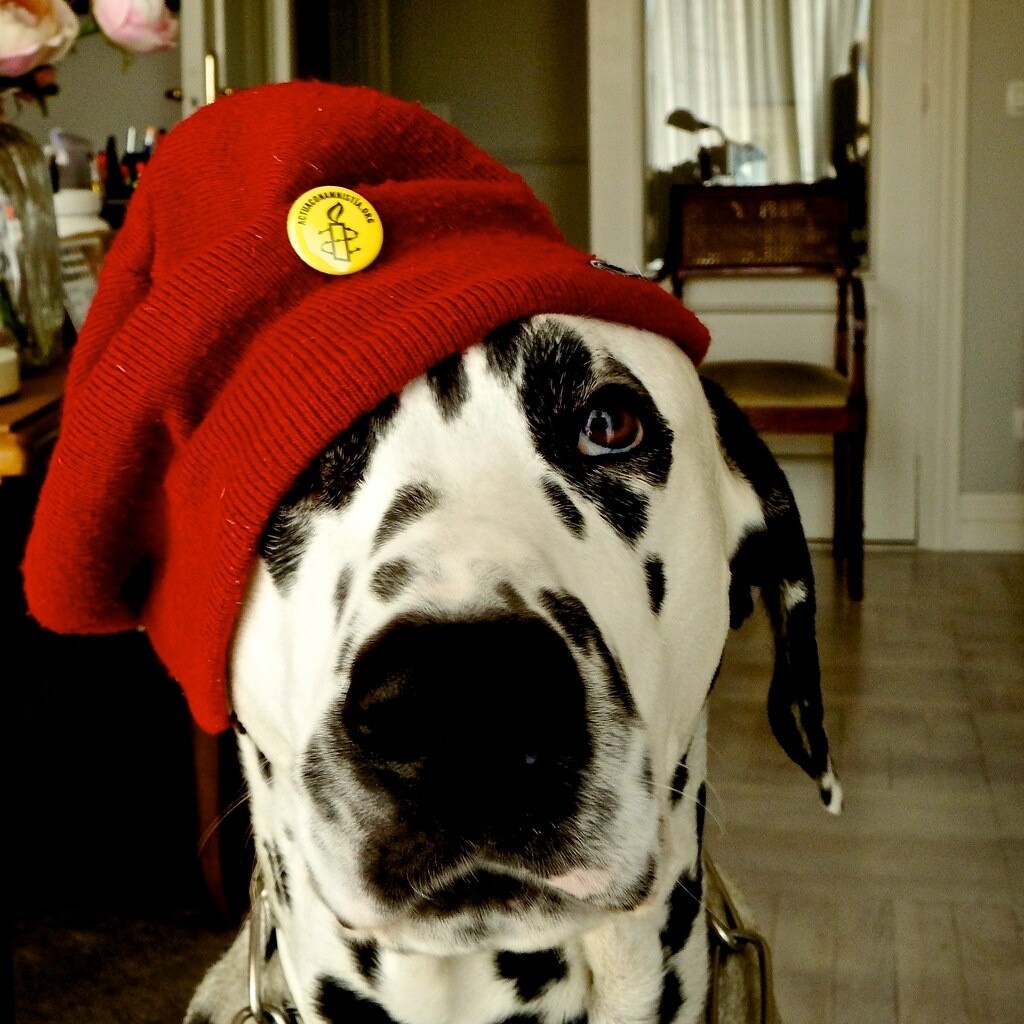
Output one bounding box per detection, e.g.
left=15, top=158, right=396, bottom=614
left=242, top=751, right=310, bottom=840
left=0, top=0, right=183, bottom=116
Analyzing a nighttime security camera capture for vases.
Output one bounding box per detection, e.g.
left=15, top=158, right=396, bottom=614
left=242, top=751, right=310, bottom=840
left=0, top=93, right=86, bottom=380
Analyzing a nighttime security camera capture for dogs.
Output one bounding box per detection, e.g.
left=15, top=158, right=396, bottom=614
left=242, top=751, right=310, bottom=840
left=178, top=310, right=844, bottom=1024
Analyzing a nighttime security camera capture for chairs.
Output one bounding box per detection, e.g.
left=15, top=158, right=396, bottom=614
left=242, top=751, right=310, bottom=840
left=660, top=174, right=872, bottom=603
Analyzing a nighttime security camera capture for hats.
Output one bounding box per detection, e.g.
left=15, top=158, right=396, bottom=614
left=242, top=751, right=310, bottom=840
left=23, top=78, right=711, bottom=731
